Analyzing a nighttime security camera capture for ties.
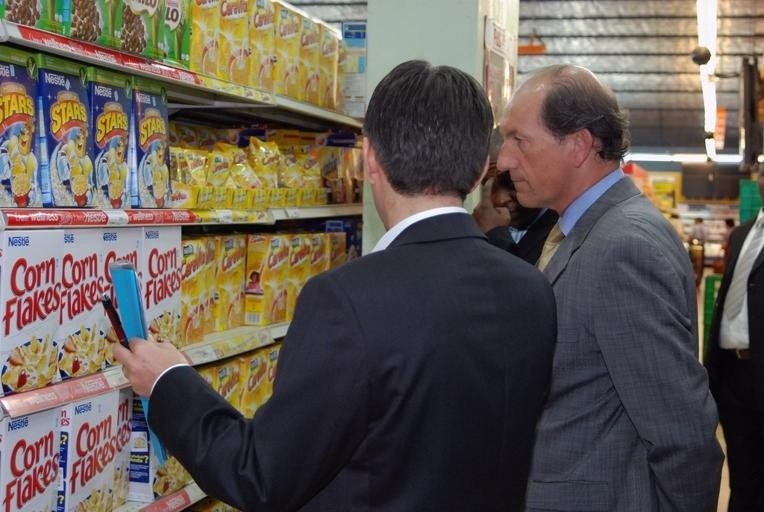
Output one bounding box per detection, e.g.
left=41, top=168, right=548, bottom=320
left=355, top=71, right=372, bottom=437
left=530, top=224, right=565, bottom=271
left=724, top=217, right=763, bottom=319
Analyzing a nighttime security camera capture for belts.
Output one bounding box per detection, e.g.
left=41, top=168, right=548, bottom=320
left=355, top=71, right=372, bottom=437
left=719, top=348, right=752, bottom=361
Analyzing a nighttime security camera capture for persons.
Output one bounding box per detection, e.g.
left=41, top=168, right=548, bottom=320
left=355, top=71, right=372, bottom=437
left=666, top=213, right=689, bottom=243
left=702, top=159, right=763, bottom=512
left=471, top=126, right=559, bottom=265
left=722, top=217, right=736, bottom=251
left=110, top=60, right=558, bottom=511
left=691, top=217, right=705, bottom=244
left=495, top=63, right=725, bottom=512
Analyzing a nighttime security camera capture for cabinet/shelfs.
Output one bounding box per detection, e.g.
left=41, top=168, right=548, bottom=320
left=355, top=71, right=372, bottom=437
left=0, top=18, right=364, bottom=512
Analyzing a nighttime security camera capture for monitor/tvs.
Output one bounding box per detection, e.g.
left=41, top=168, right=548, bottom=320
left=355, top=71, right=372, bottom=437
left=742, top=58, right=761, bottom=163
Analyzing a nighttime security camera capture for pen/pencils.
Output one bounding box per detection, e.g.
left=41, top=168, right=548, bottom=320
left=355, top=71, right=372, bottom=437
left=100, top=293, right=128, bottom=348
